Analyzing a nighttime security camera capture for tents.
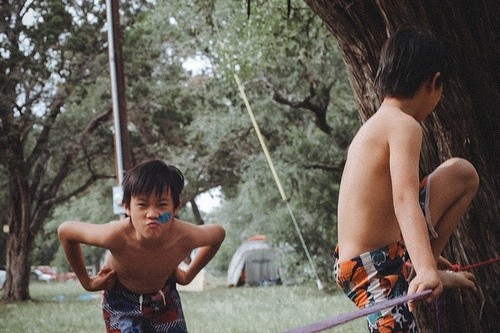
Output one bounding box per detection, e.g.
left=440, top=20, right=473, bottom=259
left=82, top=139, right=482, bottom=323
left=227, top=234, right=298, bottom=289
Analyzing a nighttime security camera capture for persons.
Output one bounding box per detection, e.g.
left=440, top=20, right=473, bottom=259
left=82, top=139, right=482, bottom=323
left=58, top=161, right=224, bottom=333
left=334, top=23, right=479, bottom=333
